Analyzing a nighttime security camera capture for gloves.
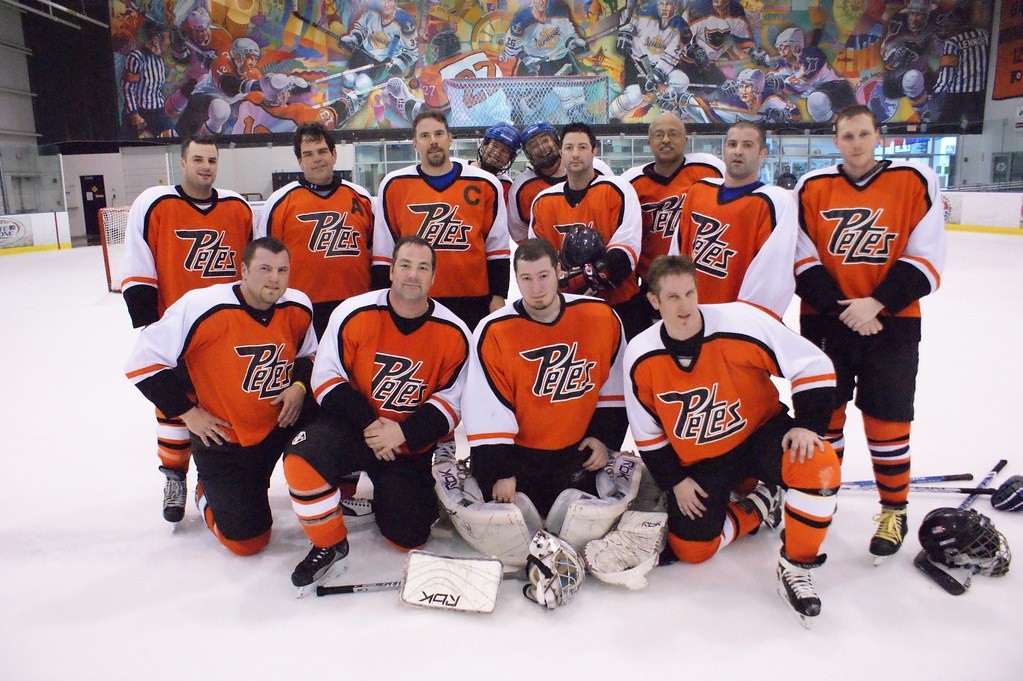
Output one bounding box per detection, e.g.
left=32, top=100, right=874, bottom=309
left=582, top=254, right=620, bottom=296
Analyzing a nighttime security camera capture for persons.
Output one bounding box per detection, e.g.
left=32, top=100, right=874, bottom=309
left=622, top=255, right=841, bottom=631
left=124, top=236, right=378, bottom=555
left=283, top=237, right=476, bottom=600
left=371, top=112, right=510, bottom=334
left=459, top=123, right=514, bottom=206
left=622, top=113, right=729, bottom=324
left=792, top=103, right=947, bottom=568
left=462, top=238, right=633, bottom=522
left=667, top=120, right=798, bottom=325
left=256, top=122, right=379, bottom=342
left=121, top=136, right=253, bottom=535
left=507, top=123, right=615, bottom=248
left=526, top=121, right=650, bottom=346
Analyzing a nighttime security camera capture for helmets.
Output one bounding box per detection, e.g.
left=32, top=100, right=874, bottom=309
left=560, top=227, right=603, bottom=269
left=520, top=120, right=561, bottom=168
left=918, top=508, right=1011, bottom=577
left=522, top=532, right=585, bottom=609
left=476, top=121, right=521, bottom=177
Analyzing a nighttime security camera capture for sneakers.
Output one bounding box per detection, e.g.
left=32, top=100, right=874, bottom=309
left=775, top=527, right=828, bottom=629
left=159, top=466, right=186, bottom=531
left=341, top=497, right=377, bottom=528
left=869, top=499, right=909, bottom=566
left=737, top=483, right=782, bottom=531
left=290, top=536, right=349, bottom=599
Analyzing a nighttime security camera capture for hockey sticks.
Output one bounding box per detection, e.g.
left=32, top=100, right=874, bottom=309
left=778, top=457, right=1015, bottom=601
left=315, top=549, right=577, bottom=597
